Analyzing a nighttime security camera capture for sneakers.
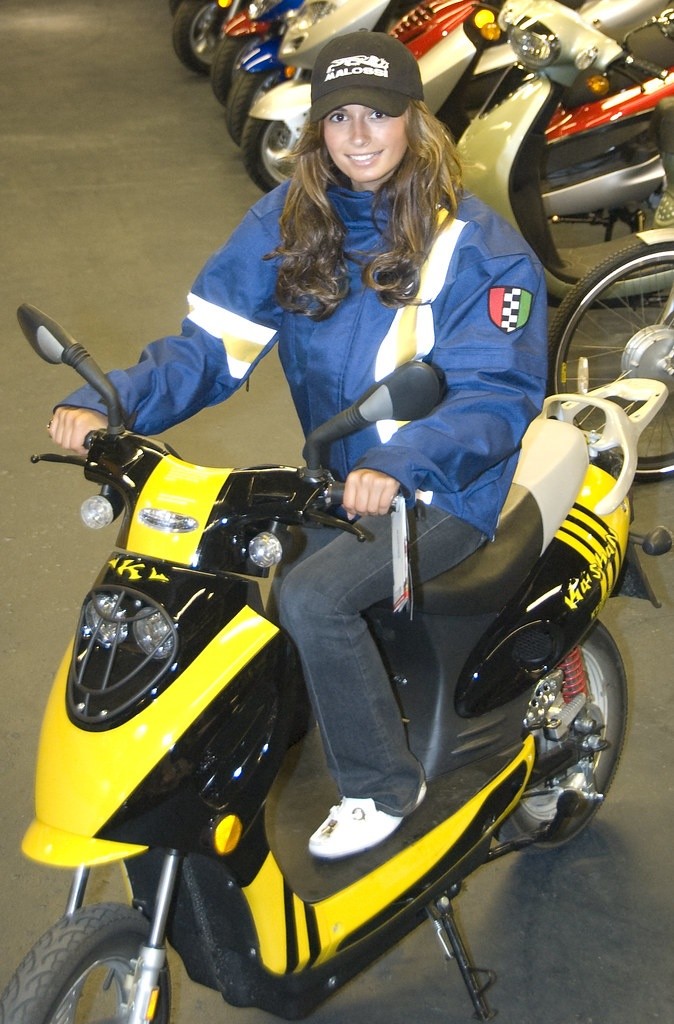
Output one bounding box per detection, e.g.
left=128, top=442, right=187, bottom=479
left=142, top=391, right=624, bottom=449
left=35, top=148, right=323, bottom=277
left=309, top=778, right=426, bottom=860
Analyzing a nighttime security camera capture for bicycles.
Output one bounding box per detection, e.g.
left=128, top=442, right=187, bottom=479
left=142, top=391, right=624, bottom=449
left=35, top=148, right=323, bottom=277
left=544, top=227, right=673, bottom=483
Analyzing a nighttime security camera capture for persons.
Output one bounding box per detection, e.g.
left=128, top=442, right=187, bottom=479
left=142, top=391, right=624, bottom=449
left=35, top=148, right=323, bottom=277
left=49, top=31, right=552, bottom=862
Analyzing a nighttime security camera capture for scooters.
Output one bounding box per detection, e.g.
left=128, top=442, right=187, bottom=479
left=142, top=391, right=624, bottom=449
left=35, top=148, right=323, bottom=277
left=169, top=0, right=674, bottom=193
left=0, top=302, right=672, bottom=1024
left=451, top=1, right=674, bottom=309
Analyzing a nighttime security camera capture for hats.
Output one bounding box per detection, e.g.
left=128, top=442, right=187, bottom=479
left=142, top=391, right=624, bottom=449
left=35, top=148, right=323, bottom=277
left=310, top=28, right=424, bottom=124
